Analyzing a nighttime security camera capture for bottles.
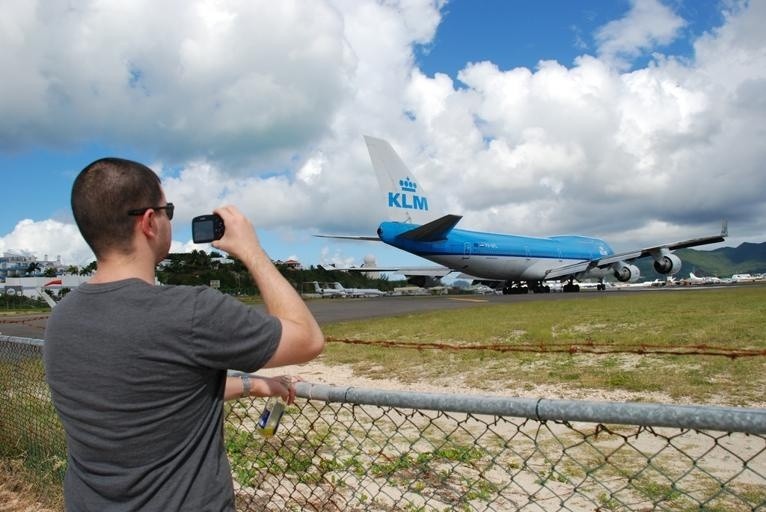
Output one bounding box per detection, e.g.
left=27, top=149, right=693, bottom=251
left=253, top=373, right=292, bottom=440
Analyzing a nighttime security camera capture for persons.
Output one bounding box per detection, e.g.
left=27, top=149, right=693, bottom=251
left=43, top=155, right=325, bottom=510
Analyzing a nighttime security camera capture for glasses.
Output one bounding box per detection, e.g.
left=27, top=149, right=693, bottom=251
left=127, top=202, right=174, bottom=221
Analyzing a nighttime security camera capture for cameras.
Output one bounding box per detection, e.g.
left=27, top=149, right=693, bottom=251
left=192, top=215, right=225, bottom=244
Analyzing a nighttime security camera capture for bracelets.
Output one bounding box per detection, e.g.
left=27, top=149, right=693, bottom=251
left=233, top=370, right=254, bottom=401
